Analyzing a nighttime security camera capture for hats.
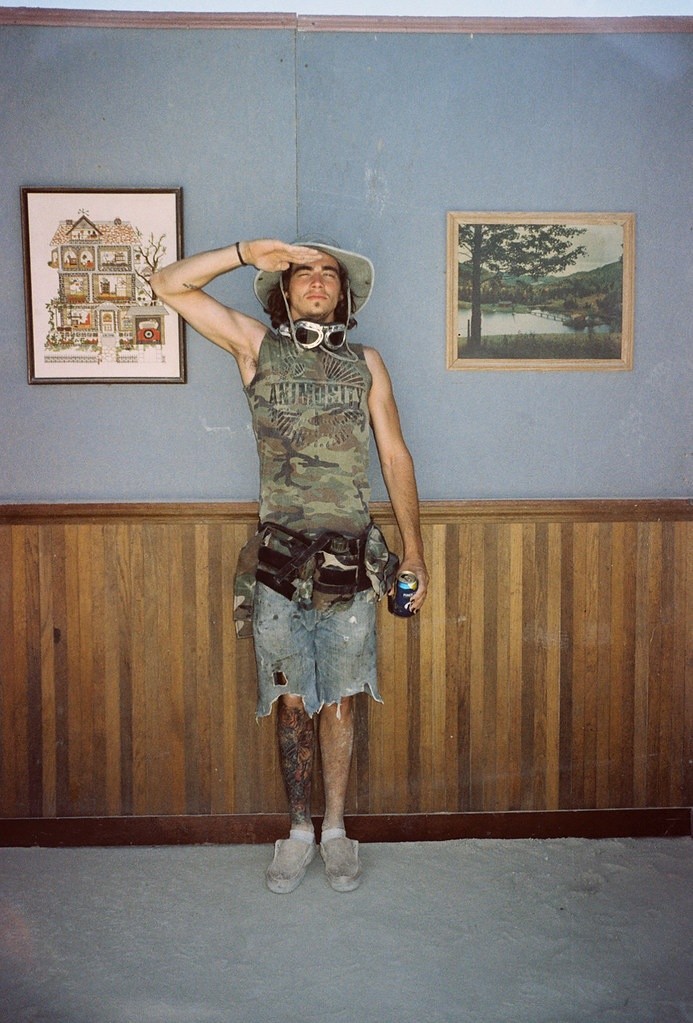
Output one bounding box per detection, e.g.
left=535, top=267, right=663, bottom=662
left=253, top=234, right=374, bottom=318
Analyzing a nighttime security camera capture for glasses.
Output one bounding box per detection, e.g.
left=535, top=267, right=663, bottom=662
left=287, top=319, right=347, bottom=351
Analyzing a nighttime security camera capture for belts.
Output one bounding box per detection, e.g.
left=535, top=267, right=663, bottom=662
left=258, top=546, right=356, bottom=587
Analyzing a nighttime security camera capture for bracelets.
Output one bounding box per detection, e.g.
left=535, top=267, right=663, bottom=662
left=234, top=242, right=248, bottom=268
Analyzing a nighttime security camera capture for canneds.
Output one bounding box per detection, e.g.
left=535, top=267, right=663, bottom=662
left=393, top=571, right=417, bottom=616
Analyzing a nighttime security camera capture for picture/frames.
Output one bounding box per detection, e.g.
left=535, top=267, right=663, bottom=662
left=18, top=184, right=187, bottom=385
left=448, top=211, right=632, bottom=371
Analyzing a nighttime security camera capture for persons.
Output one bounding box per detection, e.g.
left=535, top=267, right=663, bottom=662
left=152, top=236, right=432, bottom=894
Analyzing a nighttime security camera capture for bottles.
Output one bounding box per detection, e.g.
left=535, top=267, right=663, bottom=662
left=323, top=537, right=354, bottom=556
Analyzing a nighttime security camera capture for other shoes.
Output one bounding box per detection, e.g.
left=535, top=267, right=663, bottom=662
left=267, top=838, right=317, bottom=894
left=319, top=836, right=360, bottom=891
